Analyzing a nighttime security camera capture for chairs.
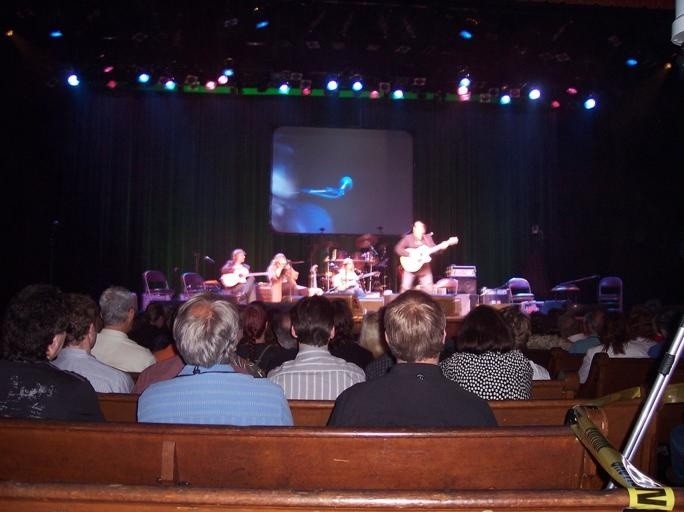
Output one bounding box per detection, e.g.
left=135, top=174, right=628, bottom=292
left=508, top=277, right=535, bottom=306
left=596, top=277, right=625, bottom=315
left=141, top=270, right=174, bottom=312
left=183, top=273, right=205, bottom=299
left=434, top=278, right=459, bottom=295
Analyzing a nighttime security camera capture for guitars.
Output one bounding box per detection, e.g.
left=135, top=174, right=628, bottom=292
left=332, top=272, right=381, bottom=292
left=223, top=271, right=269, bottom=291
left=400, top=236, right=458, bottom=274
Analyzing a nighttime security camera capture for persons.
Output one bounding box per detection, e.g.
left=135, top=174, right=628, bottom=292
left=396, top=220, right=447, bottom=296
left=267, top=252, right=299, bottom=288
left=331, top=258, right=364, bottom=296
left=222, top=248, right=256, bottom=304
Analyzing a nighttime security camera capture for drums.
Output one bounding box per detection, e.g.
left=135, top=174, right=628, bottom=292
left=377, top=255, right=392, bottom=270
left=352, top=252, right=367, bottom=272
left=367, top=253, right=376, bottom=267
left=332, top=251, right=346, bottom=270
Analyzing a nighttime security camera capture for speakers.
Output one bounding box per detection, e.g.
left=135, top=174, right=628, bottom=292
left=446, top=266, right=478, bottom=295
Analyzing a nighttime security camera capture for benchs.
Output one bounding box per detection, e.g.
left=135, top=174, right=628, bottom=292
left=486, top=346, right=684, bottom=489
left=287, top=399, right=334, bottom=428
left=96, top=392, right=141, bottom=421
left=1, top=413, right=684, bottom=511
left=126, top=371, right=142, bottom=385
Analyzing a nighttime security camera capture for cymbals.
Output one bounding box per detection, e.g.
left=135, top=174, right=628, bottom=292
left=377, top=241, right=389, bottom=251
left=308, top=245, right=325, bottom=253
left=320, top=241, right=337, bottom=251
left=354, top=235, right=379, bottom=249
